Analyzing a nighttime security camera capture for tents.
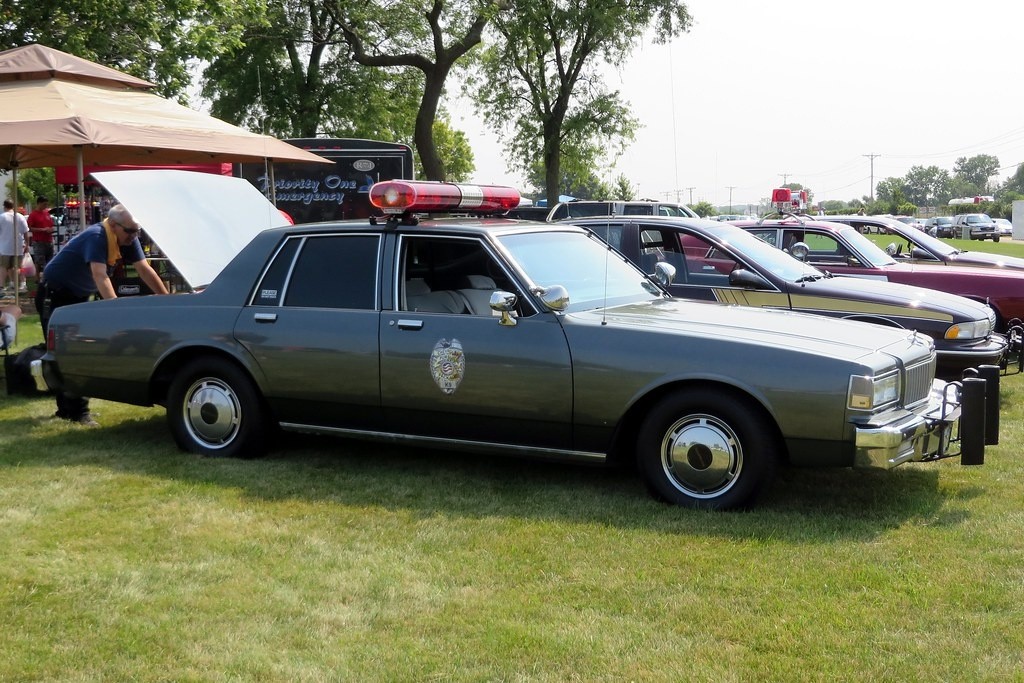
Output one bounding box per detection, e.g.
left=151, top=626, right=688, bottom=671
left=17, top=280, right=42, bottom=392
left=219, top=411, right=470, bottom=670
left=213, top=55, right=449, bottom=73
left=0, top=44, right=335, bottom=344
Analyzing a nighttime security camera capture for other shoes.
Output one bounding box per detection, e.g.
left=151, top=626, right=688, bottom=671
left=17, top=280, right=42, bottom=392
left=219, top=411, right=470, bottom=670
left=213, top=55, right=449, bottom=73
left=55, top=410, right=101, bottom=428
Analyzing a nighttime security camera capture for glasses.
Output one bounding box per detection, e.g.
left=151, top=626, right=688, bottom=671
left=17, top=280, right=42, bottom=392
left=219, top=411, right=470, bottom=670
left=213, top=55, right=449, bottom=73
left=113, top=221, right=142, bottom=234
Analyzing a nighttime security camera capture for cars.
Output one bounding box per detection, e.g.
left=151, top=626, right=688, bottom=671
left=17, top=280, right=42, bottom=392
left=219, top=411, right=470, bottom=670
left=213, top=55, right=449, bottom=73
left=703, top=215, right=759, bottom=222
left=795, top=217, right=1024, bottom=274
left=563, top=217, right=1024, bottom=388
left=866, top=215, right=952, bottom=238
left=992, top=219, right=1012, bottom=237
left=31, top=222, right=998, bottom=512
left=650, top=218, right=1024, bottom=329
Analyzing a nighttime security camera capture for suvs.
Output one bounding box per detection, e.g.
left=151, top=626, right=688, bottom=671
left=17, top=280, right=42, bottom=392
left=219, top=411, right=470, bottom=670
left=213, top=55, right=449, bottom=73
left=950, top=214, right=1001, bottom=242
left=547, top=199, right=699, bottom=252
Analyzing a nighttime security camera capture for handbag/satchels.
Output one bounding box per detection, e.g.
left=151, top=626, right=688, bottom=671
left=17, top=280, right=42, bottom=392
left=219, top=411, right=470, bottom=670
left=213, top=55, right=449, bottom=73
left=20, top=252, right=36, bottom=277
left=5, top=343, right=54, bottom=398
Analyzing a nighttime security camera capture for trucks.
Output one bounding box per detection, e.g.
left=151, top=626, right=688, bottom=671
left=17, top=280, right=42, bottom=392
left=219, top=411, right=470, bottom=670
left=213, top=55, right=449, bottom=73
left=948, top=195, right=996, bottom=206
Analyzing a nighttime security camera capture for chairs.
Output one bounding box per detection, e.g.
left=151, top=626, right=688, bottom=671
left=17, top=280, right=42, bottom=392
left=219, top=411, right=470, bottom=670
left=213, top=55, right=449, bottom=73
left=457, top=275, right=503, bottom=315
left=406, top=278, right=464, bottom=314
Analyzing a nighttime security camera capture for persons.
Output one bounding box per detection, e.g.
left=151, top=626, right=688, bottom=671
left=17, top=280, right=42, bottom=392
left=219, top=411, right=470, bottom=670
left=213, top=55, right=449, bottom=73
left=0, top=195, right=168, bottom=427
left=816, top=208, right=824, bottom=238
left=857, top=208, right=864, bottom=235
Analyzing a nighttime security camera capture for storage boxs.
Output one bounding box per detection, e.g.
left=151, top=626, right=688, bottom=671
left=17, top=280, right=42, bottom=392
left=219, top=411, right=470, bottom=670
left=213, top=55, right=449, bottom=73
left=5, top=354, right=59, bottom=396
